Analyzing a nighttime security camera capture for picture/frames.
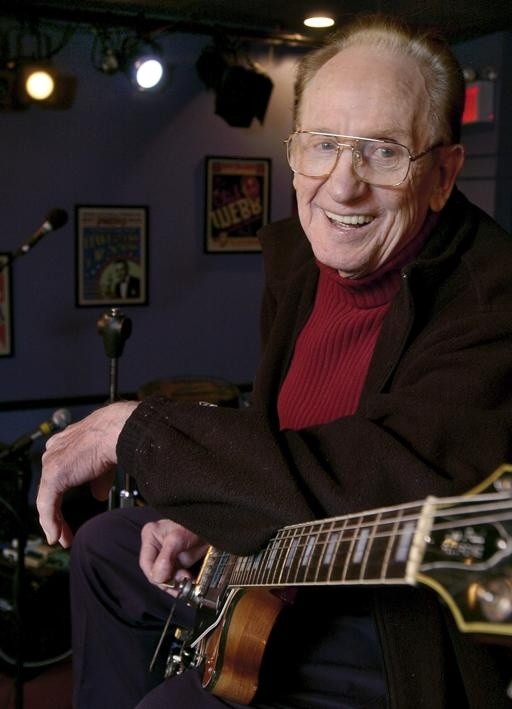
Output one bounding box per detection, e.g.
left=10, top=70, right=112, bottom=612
left=203, top=154, right=273, bottom=254
left=1, top=252, right=15, bottom=358
left=72, top=204, right=152, bottom=309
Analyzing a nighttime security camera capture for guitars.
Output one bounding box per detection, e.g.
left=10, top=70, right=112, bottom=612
left=147, top=463, right=512, bottom=708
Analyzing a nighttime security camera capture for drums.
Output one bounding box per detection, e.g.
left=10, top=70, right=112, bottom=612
left=0, top=505, right=72, bottom=676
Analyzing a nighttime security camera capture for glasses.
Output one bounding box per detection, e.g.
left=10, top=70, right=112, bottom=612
left=284, top=131, right=443, bottom=186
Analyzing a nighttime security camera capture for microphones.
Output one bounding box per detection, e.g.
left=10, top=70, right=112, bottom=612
left=12, top=408, right=72, bottom=454
left=21, top=207, right=69, bottom=254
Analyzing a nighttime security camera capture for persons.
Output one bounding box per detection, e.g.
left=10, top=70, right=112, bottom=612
left=227, top=175, right=262, bottom=237
left=31, top=11, right=512, bottom=709
left=104, top=257, right=142, bottom=300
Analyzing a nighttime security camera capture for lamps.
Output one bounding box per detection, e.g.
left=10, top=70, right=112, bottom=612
left=14, top=3, right=168, bottom=102
